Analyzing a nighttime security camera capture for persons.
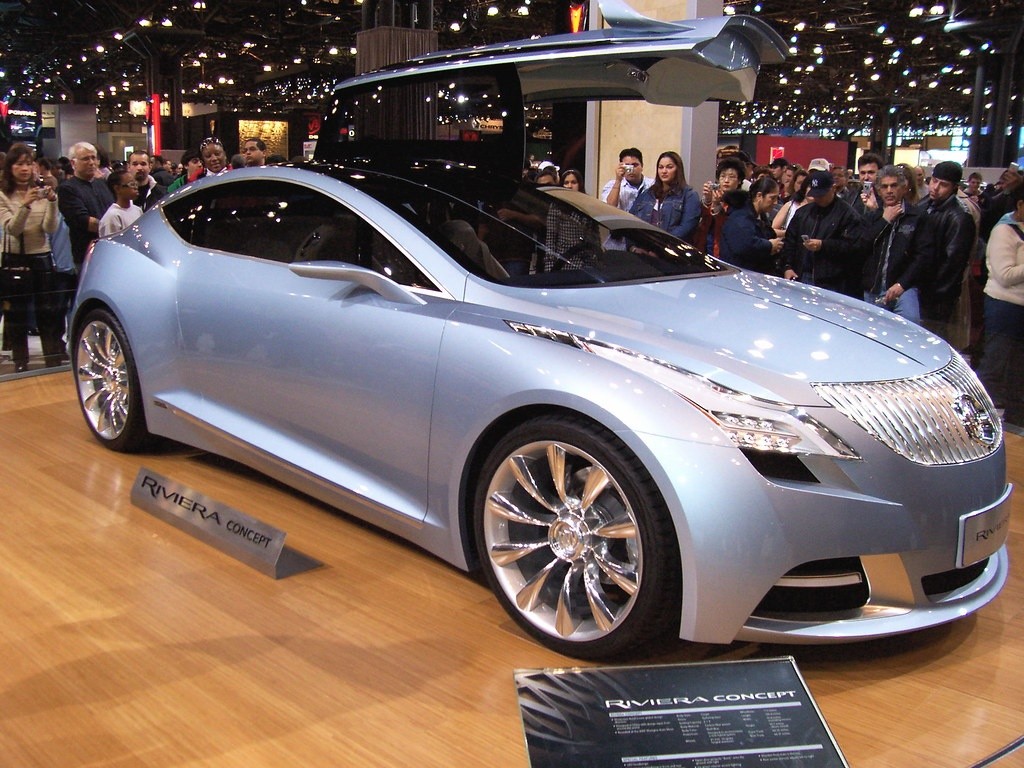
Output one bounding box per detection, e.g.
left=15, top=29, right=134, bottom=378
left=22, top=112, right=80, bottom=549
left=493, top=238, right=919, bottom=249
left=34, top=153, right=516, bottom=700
left=626, top=152, right=701, bottom=257
left=495, top=154, right=602, bottom=272
left=0, top=137, right=267, bottom=372
left=692, top=157, right=746, bottom=259
left=721, top=153, right=1024, bottom=423
left=601, top=148, right=656, bottom=252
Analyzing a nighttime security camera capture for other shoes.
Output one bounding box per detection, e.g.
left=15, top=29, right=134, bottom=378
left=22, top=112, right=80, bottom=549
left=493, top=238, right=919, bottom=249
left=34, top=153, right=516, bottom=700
left=14, top=361, right=27, bottom=373
left=969, top=314, right=984, bottom=329
left=45, top=354, right=61, bottom=368
left=962, top=340, right=985, bottom=354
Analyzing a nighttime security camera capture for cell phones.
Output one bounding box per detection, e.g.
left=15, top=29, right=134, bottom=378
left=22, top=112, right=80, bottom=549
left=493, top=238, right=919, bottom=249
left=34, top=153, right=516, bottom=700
left=801, top=235, right=810, bottom=243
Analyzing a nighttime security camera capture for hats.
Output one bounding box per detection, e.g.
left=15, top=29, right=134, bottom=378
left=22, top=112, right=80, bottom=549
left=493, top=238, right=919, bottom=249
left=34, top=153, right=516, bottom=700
left=807, top=171, right=834, bottom=198
left=932, top=162, right=969, bottom=189
left=806, top=158, right=831, bottom=173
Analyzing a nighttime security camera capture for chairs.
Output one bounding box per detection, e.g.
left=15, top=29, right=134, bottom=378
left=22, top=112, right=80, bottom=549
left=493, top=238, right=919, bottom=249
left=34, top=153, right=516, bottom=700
left=292, top=210, right=357, bottom=262
left=443, top=220, right=506, bottom=281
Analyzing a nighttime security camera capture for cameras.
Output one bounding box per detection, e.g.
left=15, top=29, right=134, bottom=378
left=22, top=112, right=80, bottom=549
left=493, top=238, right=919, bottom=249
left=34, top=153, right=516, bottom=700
left=875, top=296, right=888, bottom=305
left=864, top=182, right=872, bottom=197
left=36, top=189, right=50, bottom=199
left=623, top=165, right=634, bottom=173
left=710, top=184, right=720, bottom=190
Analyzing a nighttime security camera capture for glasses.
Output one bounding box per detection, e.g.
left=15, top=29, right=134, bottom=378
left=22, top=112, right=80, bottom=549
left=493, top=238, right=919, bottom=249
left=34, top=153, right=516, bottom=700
left=719, top=173, right=739, bottom=181
left=116, top=181, right=139, bottom=189
left=199, top=136, right=222, bottom=153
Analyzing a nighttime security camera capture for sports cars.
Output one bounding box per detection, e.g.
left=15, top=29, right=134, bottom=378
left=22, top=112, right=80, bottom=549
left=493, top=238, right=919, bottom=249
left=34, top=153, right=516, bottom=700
left=62, top=0, right=1013, bottom=662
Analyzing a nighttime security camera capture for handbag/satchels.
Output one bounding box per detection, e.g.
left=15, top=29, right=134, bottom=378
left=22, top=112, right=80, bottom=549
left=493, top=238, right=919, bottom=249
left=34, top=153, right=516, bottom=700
left=0, top=220, right=44, bottom=304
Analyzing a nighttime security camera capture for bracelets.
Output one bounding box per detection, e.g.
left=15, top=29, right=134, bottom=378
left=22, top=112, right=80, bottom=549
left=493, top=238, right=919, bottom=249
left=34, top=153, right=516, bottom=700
left=702, top=196, right=712, bottom=208
left=710, top=202, right=723, bottom=216
